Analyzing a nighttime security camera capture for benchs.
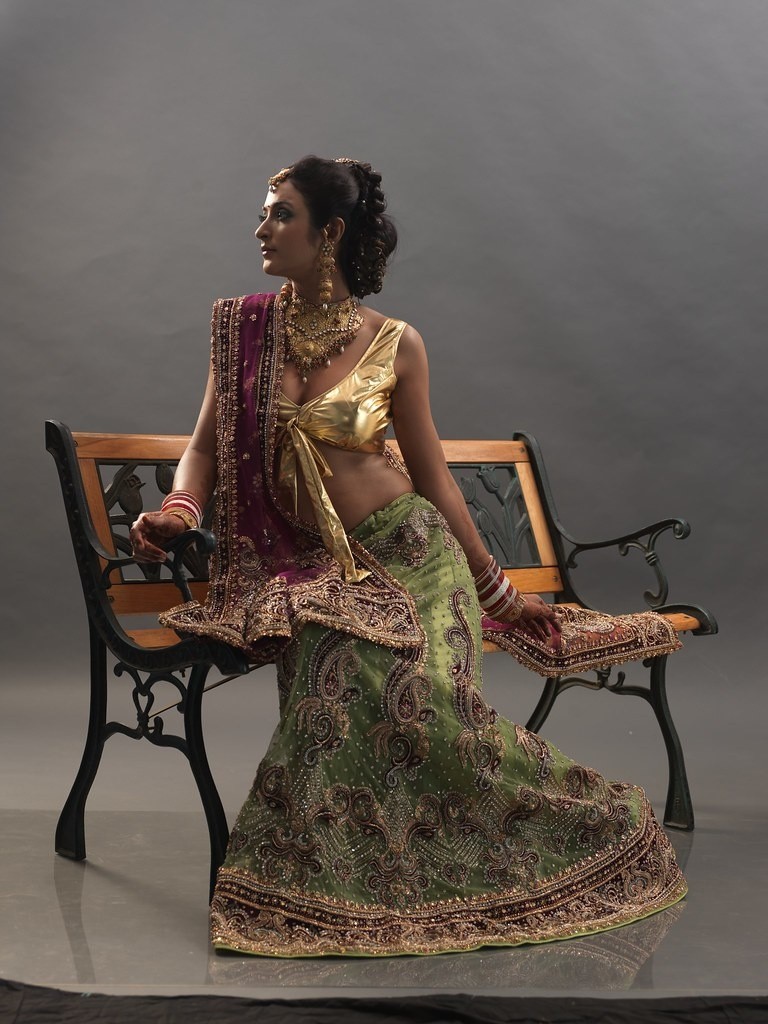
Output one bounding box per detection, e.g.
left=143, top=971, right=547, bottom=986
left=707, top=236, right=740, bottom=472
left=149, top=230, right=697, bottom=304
left=43, top=419, right=718, bottom=909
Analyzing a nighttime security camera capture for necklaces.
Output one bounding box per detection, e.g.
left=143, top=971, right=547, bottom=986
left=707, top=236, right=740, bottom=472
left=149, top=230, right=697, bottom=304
left=277, top=290, right=364, bottom=384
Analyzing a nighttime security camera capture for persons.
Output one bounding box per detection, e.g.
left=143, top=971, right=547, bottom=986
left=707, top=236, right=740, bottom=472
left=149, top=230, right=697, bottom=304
left=128, top=155, right=564, bottom=949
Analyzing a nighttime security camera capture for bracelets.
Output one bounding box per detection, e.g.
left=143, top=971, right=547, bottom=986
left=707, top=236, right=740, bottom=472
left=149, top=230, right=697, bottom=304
left=161, top=489, right=203, bottom=533
left=473, top=553, right=527, bottom=626
